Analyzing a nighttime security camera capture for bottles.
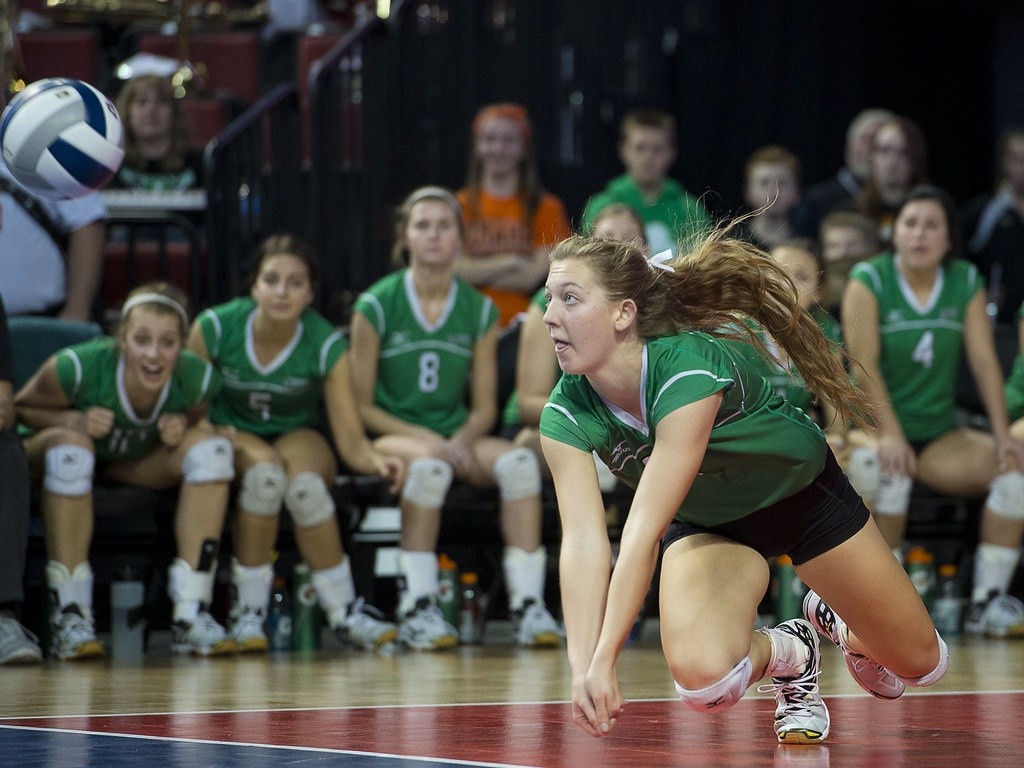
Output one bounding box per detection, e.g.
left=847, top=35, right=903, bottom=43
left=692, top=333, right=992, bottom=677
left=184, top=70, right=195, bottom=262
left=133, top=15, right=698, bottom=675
left=437, top=554, right=458, bottom=630
left=772, top=553, right=809, bottom=626
left=110, top=563, right=145, bottom=666
left=264, top=577, right=293, bottom=651
left=458, top=573, right=483, bottom=645
left=293, top=562, right=320, bottom=650
left=906, top=544, right=937, bottom=612
left=930, top=564, right=960, bottom=636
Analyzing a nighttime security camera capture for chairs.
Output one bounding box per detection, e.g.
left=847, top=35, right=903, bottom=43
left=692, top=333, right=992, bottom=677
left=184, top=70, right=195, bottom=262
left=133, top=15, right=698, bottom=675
left=13, top=322, right=496, bottom=638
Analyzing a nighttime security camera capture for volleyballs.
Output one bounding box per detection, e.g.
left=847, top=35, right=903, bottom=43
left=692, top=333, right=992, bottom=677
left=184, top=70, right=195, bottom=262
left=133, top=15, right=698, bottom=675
left=0, top=76, right=126, bottom=200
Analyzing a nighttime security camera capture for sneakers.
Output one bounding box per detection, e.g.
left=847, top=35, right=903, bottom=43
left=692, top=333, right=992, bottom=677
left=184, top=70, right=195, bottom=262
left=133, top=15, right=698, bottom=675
left=171, top=610, right=237, bottom=656
left=511, top=595, right=563, bottom=648
left=0, top=617, right=43, bottom=665
left=47, top=602, right=105, bottom=660
left=803, top=589, right=906, bottom=702
left=332, top=598, right=399, bottom=651
left=964, top=587, right=1024, bottom=639
left=757, top=618, right=830, bottom=744
left=397, top=592, right=459, bottom=652
left=227, top=605, right=269, bottom=652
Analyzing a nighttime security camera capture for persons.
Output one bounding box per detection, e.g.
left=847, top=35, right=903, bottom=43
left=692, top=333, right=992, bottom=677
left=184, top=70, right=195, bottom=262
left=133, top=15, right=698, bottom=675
left=540, top=215, right=953, bottom=743
left=0, top=1, right=1023, bottom=660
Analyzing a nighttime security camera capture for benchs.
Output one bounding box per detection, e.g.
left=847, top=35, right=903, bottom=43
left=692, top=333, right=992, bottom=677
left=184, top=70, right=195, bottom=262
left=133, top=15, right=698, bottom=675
left=0, top=0, right=428, bottom=338
